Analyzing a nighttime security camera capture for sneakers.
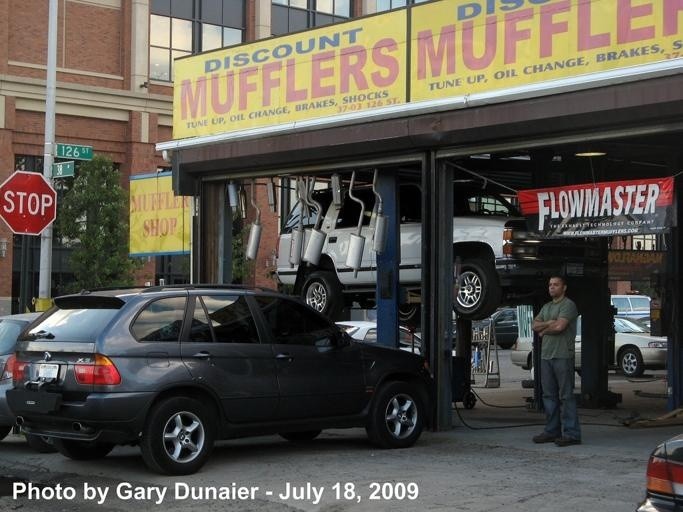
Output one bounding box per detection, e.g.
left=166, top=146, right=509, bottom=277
left=532, top=432, right=562, bottom=444
left=555, top=436, right=582, bottom=447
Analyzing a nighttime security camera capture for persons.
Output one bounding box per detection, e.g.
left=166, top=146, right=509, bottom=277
left=531, top=275, right=581, bottom=446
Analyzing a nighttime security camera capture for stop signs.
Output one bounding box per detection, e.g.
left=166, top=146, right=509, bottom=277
left=0, top=170, right=57, bottom=237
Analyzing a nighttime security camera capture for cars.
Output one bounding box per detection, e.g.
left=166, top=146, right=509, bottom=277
left=472, top=294, right=666, bottom=377
left=639, top=433, right=683, bottom=512
left=276, top=188, right=601, bottom=325
